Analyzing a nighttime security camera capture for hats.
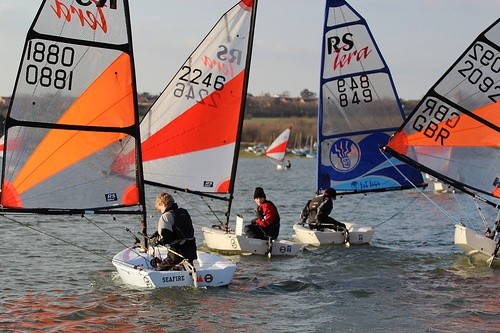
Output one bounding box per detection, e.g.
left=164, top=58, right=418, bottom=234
left=325, top=188, right=336, bottom=200
left=254, top=187, right=265, bottom=199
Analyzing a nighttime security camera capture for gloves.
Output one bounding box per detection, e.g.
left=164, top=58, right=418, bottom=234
left=298, top=218, right=306, bottom=225
left=251, top=220, right=256, bottom=224
left=149, top=236, right=158, bottom=246
left=316, top=219, right=324, bottom=226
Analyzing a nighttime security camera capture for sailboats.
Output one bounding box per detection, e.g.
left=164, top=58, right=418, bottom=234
left=1, top=0, right=236, bottom=292
left=104, top=0, right=300, bottom=259
left=414, top=147, right=462, bottom=191
left=289, top=0, right=429, bottom=246
left=245, top=124, right=318, bottom=170
left=381, top=18, right=499, bottom=258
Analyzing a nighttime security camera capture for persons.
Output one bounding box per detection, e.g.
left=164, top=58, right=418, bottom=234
left=244, top=187, right=280, bottom=240
left=300, top=188, right=346, bottom=231
left=484, top=219, right=500, bottom=243
left=151, top=192, right=197, bottom=271
left=286, top=160, right=291, bottom=169
